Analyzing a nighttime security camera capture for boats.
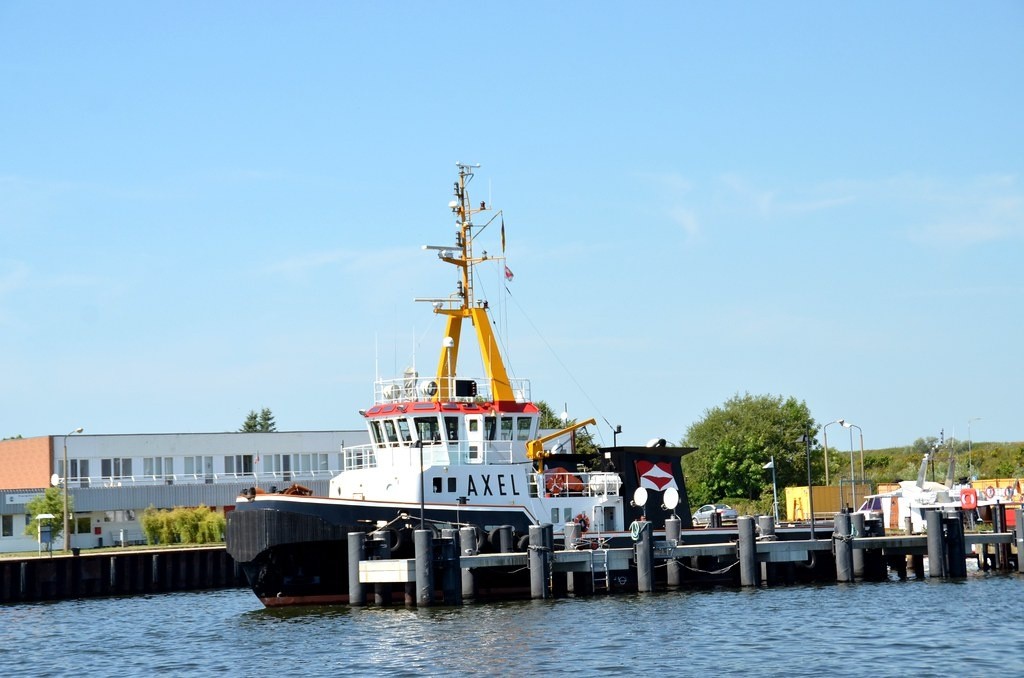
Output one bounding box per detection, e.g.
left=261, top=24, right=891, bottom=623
left=224, top=160, right=703, bottom=608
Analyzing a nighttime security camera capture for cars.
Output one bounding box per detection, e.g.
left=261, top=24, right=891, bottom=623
left=692, top=504, right=737, bottom=529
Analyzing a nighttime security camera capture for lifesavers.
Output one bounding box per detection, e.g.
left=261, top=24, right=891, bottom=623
left=1004, top=486, right=1015, bottom=498
left=985, top=486, right=996, bottom=500
left=546, top=474, right=564, bottom=493
left=574, top=513, right=590, bottom=534
left=385, top=521, right=531, bottom=552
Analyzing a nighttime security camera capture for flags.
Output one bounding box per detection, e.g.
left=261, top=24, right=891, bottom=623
left=635, top=461, right=681, bottom=492
left=504, top=265, right=514, bottom=282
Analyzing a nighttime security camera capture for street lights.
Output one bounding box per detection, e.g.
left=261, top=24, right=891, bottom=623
left=794, top=426, right=815, bottom=540
left=824, top=419, right=845, bottom=486
left=844, top=422, right=865, bottom=486
left=763, top=454, right=781, bottom=526
left=64, top=427, right=84, bottom=553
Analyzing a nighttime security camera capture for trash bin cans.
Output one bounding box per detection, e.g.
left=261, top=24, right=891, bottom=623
left=711, top=509, right=721, bottom=528
left=840, top=501, right=855, bottom=514
